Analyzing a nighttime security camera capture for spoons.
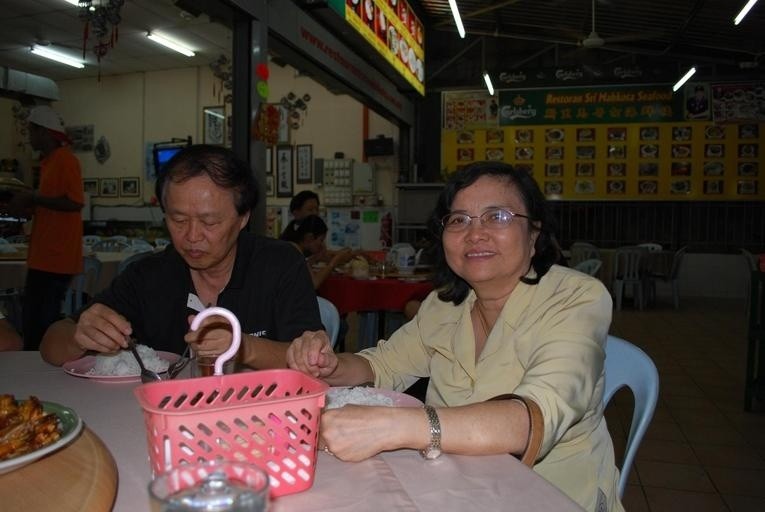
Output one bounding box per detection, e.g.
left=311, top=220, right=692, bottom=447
left=126, top=335, right=161, bottom=384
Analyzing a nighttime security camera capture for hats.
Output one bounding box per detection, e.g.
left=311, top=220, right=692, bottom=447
left=27, top=106, right=69, bottom=141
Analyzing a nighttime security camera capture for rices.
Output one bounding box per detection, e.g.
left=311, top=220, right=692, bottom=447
left=90, top=343, right=170, bottom=378
left=321, top=387, right=396, bottom=409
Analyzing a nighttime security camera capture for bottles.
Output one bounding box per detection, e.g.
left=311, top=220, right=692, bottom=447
left=378, top=254, right=386, bottom=279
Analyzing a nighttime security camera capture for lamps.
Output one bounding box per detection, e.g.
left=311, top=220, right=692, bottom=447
left=146, top=29, right=196, bottom=57
left=31, top=40, right=88, bottom=72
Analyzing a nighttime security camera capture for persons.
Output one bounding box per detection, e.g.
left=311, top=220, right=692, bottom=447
left=39, top=143, right=328, bottom=370
left=279, top=215, right=354, bottom=293
left=287, top=158, right=626, bottom=512
left=290, top=189, right=320, bottom=217
left=11, top=105, right=84, bottom=349
left=403, top=300, right=421, bottom=318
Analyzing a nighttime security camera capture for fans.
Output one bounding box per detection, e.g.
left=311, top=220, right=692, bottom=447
left=561, top=0, right=649, bottom=58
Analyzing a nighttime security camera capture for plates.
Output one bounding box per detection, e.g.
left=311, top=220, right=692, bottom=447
left=63, top=350, right=183, bottom=383
left=1, top=401, right=83, bottom=475
left=326, top=387, right=425, bottom=408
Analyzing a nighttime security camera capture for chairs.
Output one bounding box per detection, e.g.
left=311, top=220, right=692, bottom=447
left=739, top=250, right=765, bottom=407
left=6, top=235, right=28, bottom=245
left=570, top=242, right=593, bottom=268
left=613, top=246, right=643, bottom=311
left=604, top=334, right=659, bottom=501
left=573, top=259, right=602, bottom=276
left=84, top=236, right=100, bottom=245
left=92, top=239, right=129, bottom=252
left=637, top=244, right=662, bottom=251
left=650, top=246, right=687, bottom=309
left=66, top=256, right=102, bottom=316
left=112, top=236, right=127, bottom=242
left=119, top=251, right=154, bottom=277
left=310, top=297, right=339, bottom=350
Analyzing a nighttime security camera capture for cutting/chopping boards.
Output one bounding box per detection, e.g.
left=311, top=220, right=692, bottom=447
left=0, top=427, right=118, bottom=511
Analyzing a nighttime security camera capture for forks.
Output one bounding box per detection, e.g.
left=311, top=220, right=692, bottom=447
left=165, top=343, right=190, bottom=380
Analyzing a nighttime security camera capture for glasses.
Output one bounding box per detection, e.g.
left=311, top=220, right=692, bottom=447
left=441, top=206, right=533, bottom=232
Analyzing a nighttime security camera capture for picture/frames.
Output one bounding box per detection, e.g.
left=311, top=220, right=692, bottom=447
left=100, top=177, right=120, bottom=198
left=120, top=177, right=140, bottom=197
left=203, top=105, right=226, bottom=146
left=83, top=177, right=100, bottom=198
left=266, top=175, right=275, bottom=197
left=266, top=145, right=273, bottom=174
left=267, top=102, right=291, bottom=144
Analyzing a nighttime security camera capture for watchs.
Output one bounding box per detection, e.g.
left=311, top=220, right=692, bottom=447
left=423, top=405, right=444, bottom=461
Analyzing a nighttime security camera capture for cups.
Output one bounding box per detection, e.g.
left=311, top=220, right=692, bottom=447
left=149, top=459, right=269, bottom=511
